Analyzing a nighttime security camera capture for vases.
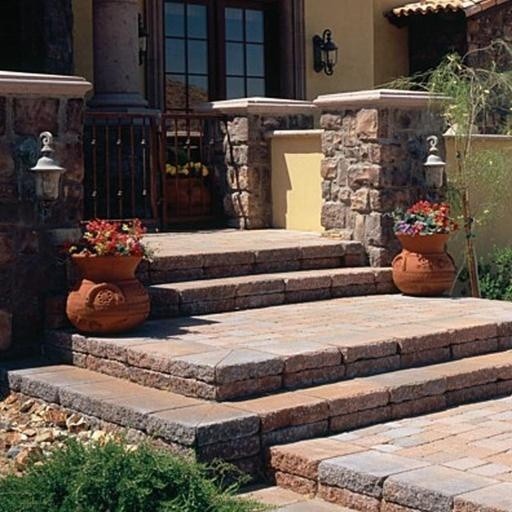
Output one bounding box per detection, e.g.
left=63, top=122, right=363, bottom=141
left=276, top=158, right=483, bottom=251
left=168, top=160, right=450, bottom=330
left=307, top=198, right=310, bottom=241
left=163, top=175, right=214, bottom=218
left=389, top=232, right=456, bottom=295
left=65, top=256, right=152, bottom=334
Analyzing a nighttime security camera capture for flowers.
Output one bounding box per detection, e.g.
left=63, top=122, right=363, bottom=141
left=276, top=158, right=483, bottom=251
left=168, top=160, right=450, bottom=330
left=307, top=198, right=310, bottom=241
left=161, top=153, right=211, bottom=180
left=389, top=201, right=455, bottom=234
left=67, top=215, right=148, bottom=258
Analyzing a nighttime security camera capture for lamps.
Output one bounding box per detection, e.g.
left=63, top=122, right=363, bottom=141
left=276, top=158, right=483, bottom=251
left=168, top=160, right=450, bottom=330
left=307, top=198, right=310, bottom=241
left=310, top=24, right=339, bottom=77
left=28, top=130, right=66, bottom=213
left=422, top=133, right=448, bottom=196
